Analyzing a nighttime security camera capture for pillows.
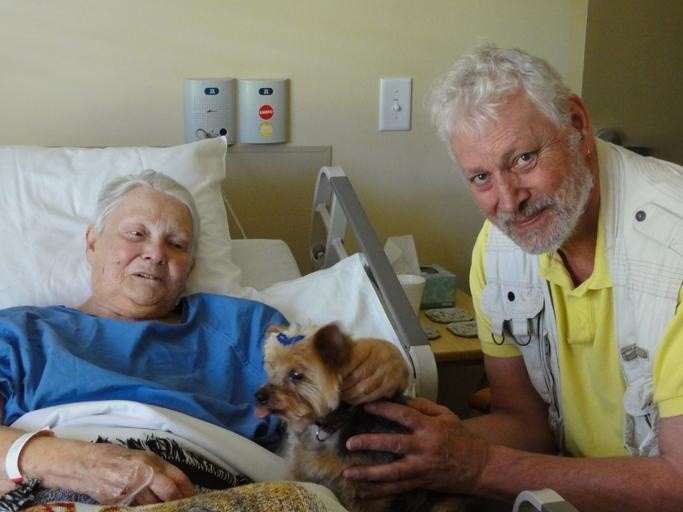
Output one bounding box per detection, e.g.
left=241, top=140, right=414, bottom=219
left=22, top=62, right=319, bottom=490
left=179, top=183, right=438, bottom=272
left=3, top=135, right=241, bottom=309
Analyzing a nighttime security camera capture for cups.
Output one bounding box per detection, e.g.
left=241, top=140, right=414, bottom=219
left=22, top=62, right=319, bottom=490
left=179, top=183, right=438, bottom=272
left=395, top=274, right=425, bottom=320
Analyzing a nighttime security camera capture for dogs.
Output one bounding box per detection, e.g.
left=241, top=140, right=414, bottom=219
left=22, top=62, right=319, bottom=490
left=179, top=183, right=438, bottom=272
left=252, top=321, right=454, bottom=511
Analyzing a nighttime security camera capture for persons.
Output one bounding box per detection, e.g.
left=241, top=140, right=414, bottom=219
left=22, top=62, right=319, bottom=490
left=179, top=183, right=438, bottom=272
left=341, top=47, right=683, bottom=512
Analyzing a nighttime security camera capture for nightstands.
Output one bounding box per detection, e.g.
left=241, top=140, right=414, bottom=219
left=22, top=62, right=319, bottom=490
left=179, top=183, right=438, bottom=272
left=416, top=286, right=489, bottom=420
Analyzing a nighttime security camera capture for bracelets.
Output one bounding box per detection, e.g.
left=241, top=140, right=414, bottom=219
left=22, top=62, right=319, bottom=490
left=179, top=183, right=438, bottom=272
left=4, top=424, right=56, bottom=487
